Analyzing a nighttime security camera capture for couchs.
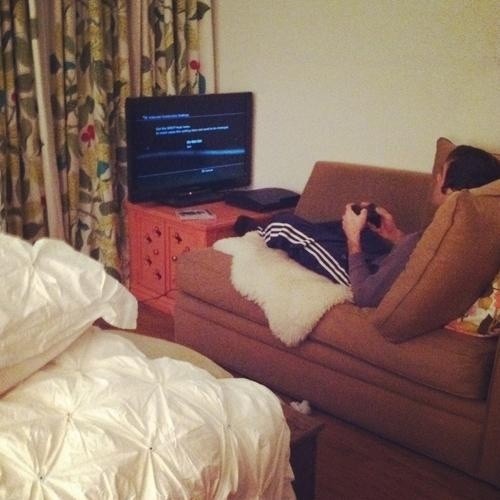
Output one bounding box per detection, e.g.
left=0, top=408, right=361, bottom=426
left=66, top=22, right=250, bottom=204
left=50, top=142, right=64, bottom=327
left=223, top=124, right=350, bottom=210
left=173, top=159, right=500, bottom=487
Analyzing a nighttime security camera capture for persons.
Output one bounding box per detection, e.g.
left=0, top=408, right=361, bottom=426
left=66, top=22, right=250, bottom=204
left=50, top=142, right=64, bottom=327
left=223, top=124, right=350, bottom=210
left=233, top=145, right=500, bottom=287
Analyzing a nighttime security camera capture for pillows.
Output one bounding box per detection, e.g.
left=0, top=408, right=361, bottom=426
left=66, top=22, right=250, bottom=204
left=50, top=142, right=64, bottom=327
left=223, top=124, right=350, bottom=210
left=443, top=271, right=500, bottom=339
left=1, top=227, right=140, bottom=394
left=428, top=138, right=500, bottom=192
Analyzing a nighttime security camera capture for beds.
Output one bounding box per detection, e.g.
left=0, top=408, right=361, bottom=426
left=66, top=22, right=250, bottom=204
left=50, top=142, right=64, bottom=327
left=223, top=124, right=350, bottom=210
left=0, top=327, right=325, bottom=500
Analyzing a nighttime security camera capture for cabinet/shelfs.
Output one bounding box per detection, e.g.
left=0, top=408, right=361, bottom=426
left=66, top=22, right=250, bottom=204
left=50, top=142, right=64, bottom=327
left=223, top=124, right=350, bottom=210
left=120, top=198, right=297, bottom=320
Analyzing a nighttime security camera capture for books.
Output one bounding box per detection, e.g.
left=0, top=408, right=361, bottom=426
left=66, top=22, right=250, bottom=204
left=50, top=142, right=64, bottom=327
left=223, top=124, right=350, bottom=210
left=175, top=208, right=216, bottom=220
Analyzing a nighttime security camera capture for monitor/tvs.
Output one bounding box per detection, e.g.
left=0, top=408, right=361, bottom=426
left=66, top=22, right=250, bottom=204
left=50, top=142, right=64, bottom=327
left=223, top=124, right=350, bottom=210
left=125, top=91, right=253, bottom=207
